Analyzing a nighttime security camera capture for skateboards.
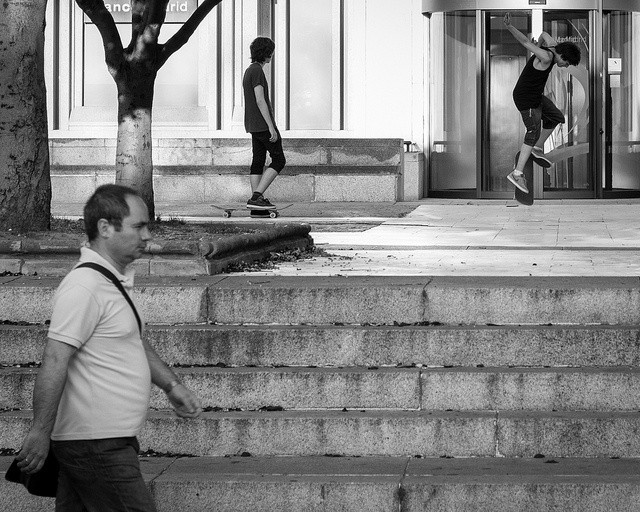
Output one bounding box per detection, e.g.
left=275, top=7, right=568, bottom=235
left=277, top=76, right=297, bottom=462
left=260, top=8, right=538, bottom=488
left=210, top=204, right=293, bottom=218
left=514, top=150, right=533, bottom=205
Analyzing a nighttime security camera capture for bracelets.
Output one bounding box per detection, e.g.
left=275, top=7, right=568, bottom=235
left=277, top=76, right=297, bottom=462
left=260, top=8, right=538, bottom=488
left=163, top=379, right=182, bottom=392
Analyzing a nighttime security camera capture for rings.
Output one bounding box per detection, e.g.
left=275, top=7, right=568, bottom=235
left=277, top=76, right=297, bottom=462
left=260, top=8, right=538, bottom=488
left=22, top=457, right=33, bottom=466
left=271, top=138, right=275, bottom=141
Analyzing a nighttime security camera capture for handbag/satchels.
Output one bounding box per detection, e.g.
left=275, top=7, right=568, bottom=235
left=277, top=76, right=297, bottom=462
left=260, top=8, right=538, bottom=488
left=5, top=262, right=141, bottom=497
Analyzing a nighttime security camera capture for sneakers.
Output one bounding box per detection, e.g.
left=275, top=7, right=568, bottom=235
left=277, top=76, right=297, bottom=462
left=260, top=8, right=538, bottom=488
left=507, top=170, right=529, bottom=195
left=530, top=148, right=552, bottom=169
left=246, top=195, right=276, bottom=209
left=249, top=210, right=280, bottom=218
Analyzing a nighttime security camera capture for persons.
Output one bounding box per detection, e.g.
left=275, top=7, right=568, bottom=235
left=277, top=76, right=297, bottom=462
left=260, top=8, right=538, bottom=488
left=243, top=37, right=286, bottom=217
left=503, top=11, right=581, bottom=193
left=6, top=184, right=202, bottom=511
